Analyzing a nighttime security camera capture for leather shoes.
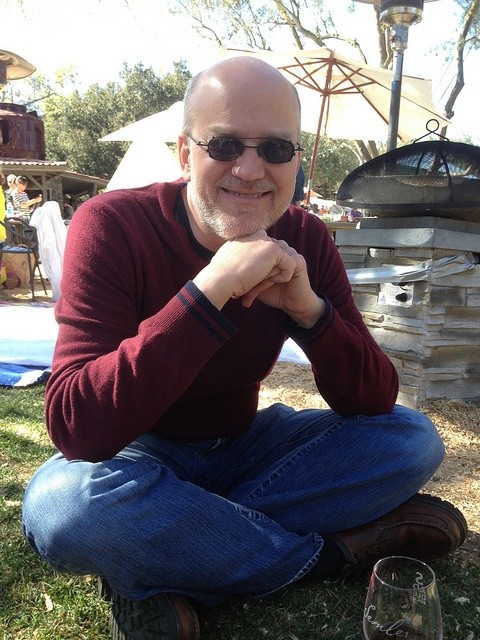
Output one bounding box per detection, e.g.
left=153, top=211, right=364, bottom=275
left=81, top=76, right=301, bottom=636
left=326, top=492, right=468, bottom=586
left=97, top=574, right=201, bottom=640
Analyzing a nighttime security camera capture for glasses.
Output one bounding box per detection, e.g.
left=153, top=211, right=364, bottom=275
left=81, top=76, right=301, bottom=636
left=186, top=131, right=304, bottom=164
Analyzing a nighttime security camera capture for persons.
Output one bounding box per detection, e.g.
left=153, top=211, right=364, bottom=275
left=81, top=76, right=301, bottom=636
left=5, top=173, right=20, bottom=230
left=309, top=204, right=362, bottom=224
left=11, top=176, right=42, bottom=224
left=0, top=167, right=7, bottom=288
left=23, top=54, right=467, bottom=640
left=289, top=163, right=305, bottom=209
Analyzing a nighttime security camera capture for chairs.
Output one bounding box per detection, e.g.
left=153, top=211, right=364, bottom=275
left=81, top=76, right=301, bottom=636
left=0, top=217, right=48, bottom=302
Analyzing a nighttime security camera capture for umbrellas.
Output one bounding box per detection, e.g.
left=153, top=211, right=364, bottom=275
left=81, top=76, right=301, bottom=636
left=228, top=47, right=452, bottom=218
left=96, top=100, right=184, bottom=188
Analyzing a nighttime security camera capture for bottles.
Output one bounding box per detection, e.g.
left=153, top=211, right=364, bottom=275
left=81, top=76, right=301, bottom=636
left=7, top=202, right=14, bottom=221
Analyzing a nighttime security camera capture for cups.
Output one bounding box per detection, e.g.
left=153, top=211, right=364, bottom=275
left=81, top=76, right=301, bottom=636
left=363, top=556, right=443, bottom=640
